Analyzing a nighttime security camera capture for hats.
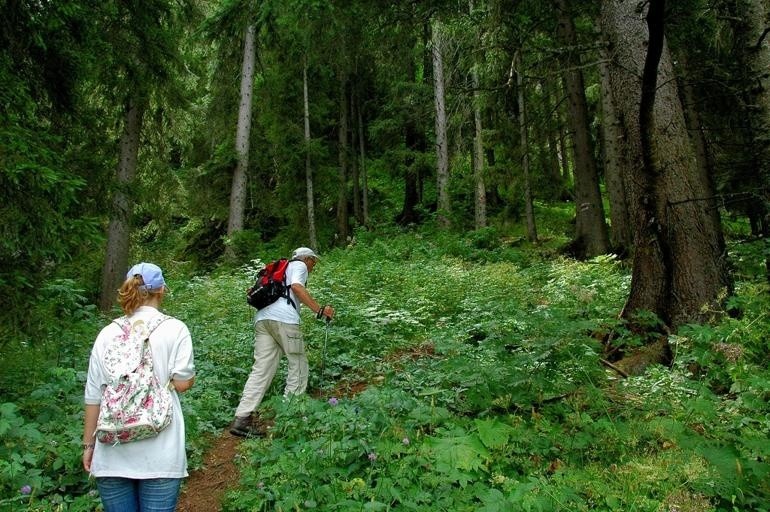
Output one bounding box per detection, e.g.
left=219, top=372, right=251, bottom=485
left=126, top=263, right=170, bottom=294
left=292, top=247, right=320, bottom=261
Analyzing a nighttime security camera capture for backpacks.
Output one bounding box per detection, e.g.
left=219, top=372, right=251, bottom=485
left=95, top=310, right=173, bottom=444
left=246, top=256, right=308, bottom=311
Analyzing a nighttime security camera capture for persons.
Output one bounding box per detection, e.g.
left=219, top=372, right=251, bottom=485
left=82, top=262, right=196, bottom=512
left=229, top=246, right=335, bottom=438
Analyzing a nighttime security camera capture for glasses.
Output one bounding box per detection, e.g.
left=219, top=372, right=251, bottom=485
left=310, top=257, right=316, bottom=265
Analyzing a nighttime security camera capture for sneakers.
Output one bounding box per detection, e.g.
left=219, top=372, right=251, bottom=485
left=230, top=414, right=266, bottom=439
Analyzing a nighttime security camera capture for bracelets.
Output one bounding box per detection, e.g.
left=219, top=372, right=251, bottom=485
left=82, top=444, right=95, bottom=449
